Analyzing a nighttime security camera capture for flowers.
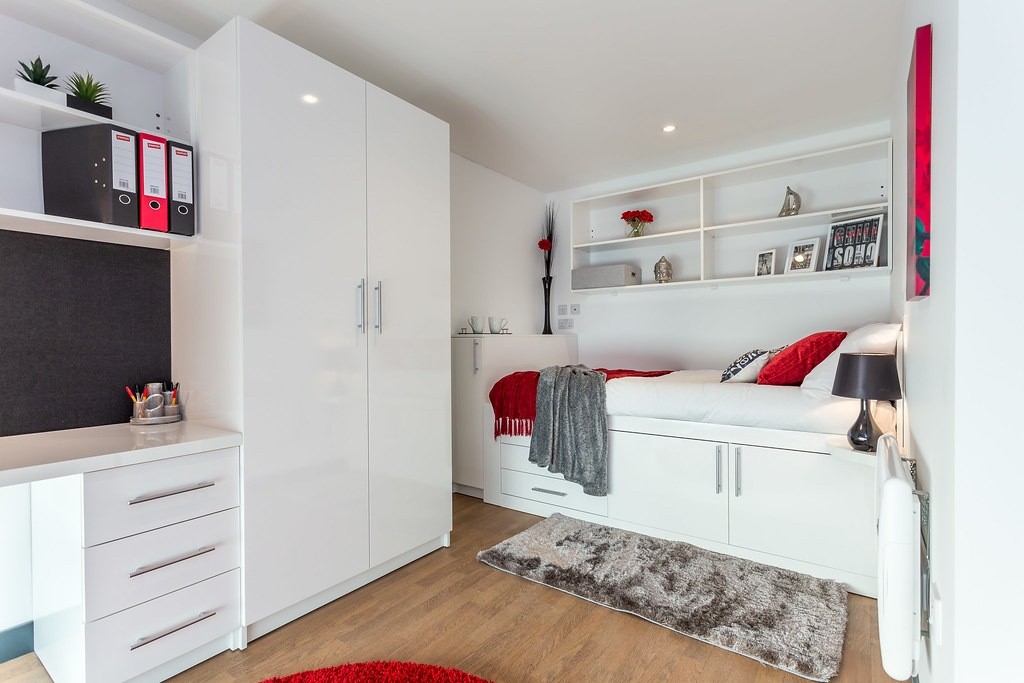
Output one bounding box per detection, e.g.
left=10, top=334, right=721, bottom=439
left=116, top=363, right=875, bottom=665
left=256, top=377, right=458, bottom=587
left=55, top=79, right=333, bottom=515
left=621, top=209, right=657, bottom=223
left=538, top=208, right=558, bottom=277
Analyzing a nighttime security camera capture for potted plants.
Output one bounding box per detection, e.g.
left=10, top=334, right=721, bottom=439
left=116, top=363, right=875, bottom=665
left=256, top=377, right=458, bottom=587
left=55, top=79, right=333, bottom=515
left=66, top=70, right=113, bottom=119
left=15, top=55, right=66, bottom=107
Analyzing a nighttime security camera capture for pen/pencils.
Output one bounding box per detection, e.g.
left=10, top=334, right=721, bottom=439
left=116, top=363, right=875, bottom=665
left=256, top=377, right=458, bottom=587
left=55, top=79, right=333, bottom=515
left=168, top=381, right=172, bottom=391
left=142, top=384, right=148, bottom=401
left=125, top=386, right=137, bottom=402
left=136, top=385, right=140, bottom=401
left=163, top=382, right=167, bottom=391
left=172, top=383, right=178, bottom=405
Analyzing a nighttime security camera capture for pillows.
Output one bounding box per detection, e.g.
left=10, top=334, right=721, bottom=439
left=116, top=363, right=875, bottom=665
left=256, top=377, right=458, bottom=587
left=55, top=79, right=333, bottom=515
left=721, top=343, right=789, bottom=384
left=754, top=332, right=847, bottom=386
left=799, top=322, right=902, bottom=389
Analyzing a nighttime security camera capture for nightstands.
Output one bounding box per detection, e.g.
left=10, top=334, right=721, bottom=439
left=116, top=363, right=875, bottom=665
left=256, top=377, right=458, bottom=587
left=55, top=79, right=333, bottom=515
left=839, top=440, right=877, bottom=467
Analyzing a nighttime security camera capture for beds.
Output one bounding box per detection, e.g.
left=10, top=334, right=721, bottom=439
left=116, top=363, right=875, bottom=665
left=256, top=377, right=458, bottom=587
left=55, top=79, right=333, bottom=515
left=483, top=369, right=878, bottom=600
left=490, top=314, right=896, bottom=449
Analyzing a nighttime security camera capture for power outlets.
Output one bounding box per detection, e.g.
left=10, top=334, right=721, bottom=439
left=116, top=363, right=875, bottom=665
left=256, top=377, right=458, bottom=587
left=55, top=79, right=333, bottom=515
left=558, top=319, right=574, bottom=329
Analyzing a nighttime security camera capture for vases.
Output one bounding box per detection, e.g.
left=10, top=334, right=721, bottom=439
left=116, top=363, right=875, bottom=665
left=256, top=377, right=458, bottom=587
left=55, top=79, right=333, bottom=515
left=626, top=221, right=646, bottom=236
left=540, top=276, right=553, bottom=335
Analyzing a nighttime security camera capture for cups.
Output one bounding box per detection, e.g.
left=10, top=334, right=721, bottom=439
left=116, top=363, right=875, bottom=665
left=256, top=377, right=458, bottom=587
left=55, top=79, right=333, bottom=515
left=164, top=405, right=179, bottom=416
left=468, top=315, right=485, bottom=333
left=163, top=391, right=177, bottom=405
left=489, top=316, right=508, bottom=333
left=133, top=402, right=145, bottom=418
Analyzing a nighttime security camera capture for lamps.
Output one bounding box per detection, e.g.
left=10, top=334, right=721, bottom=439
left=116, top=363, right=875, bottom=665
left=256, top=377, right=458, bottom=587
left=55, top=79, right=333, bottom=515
left=830, top=351, right=907, bottom=446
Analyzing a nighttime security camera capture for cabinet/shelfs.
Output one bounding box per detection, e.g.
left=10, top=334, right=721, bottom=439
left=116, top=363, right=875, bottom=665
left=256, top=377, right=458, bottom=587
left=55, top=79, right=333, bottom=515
left=569, top=135, right=894, bottom=292
left=0, top=418, right=245, bottom=683
left=0, top=0, right=202, bottom=249
left=451, top=335, right=581, bottom=502
left=608, top=430, right=843, bottom=579
left=187, top=15, right=454, bottom=649
left=495, top=429, right=609, bottom=520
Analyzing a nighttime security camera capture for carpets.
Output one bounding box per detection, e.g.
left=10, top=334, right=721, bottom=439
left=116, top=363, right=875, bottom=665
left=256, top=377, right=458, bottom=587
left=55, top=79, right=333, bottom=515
left=259, top=658, right=494, bottom=683
left=476, top=512, right=851, bottom=683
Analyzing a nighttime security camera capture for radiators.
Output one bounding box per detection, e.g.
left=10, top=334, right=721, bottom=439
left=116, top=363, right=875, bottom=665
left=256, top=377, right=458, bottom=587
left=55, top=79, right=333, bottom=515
left=876, top=431, right=921, bottom=681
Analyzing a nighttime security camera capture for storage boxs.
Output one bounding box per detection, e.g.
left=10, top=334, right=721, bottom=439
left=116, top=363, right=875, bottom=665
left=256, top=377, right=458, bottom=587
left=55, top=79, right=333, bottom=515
left=571, top=264, right=642, bottom=290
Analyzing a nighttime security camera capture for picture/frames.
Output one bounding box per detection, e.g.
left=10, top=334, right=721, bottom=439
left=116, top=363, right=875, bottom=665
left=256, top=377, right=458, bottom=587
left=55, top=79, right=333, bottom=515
left=820, top=213, right=884, bottom=273
left=754, top=249, right=776, bottom=276
left=783, top=237, right=821, bottom=274
